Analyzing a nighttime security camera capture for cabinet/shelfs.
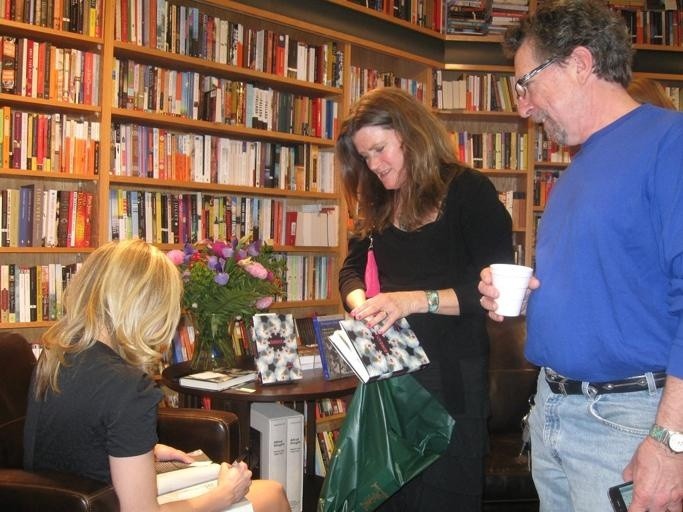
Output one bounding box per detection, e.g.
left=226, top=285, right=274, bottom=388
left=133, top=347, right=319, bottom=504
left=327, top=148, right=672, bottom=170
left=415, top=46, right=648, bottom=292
left=0, top=1, right=683, bottom=480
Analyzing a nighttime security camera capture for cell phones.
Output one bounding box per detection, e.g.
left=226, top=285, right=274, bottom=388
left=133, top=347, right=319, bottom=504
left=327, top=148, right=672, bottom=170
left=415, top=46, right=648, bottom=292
left=607, top=481, right=634, bottom=512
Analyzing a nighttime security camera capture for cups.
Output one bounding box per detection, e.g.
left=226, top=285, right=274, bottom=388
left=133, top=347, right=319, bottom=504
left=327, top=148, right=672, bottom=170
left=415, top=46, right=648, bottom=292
left=487, top=263, right=534, bottom=319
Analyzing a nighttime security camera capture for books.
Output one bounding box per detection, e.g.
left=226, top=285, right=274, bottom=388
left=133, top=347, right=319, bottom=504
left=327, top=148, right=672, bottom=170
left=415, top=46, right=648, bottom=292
left=311, top=315, right=355, bottom=382
left=1, top=1, right=683, bottom=359
left=178, top=366, right=256, bottom=393
left=329, top=319, right=429, bottom=385
left=156, top=448, right=255, bottom=512
left=251, top=394, right=352, bottom=512
left=252, top=312, right=302, bottom=385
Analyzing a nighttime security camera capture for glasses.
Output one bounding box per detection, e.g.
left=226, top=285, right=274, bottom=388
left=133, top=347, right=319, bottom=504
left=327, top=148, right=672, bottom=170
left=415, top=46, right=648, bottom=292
left=515, top=55, right=559, bottom=99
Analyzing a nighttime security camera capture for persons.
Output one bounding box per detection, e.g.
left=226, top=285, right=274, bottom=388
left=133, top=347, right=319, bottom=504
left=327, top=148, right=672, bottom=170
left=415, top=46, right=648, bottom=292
left=478, top=2, right=683, bottom=512
left=23, top=239, right=292, bottom=511
left=625, top=79, right=676, bottom=111
left=337, top=87, right=510, bottom=507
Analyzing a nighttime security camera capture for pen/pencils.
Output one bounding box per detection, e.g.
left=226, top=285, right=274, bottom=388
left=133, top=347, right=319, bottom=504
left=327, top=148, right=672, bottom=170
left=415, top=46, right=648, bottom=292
left=229, top=446, right=250, bottom=468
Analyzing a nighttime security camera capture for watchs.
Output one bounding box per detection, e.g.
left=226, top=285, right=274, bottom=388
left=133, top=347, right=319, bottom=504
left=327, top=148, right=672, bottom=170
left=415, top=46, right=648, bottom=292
left=651, top=411, right=683, bottom=460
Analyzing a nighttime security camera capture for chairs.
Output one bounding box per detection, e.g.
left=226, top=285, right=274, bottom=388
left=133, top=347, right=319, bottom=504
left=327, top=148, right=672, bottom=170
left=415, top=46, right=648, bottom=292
left=482, top=369, right=540, bottom=511
left=0, top=332, right=243, bottom=512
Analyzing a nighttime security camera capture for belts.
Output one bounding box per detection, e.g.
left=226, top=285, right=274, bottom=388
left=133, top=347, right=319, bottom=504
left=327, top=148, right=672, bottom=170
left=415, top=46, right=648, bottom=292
left=545, top=370, right=666, bottom=395
